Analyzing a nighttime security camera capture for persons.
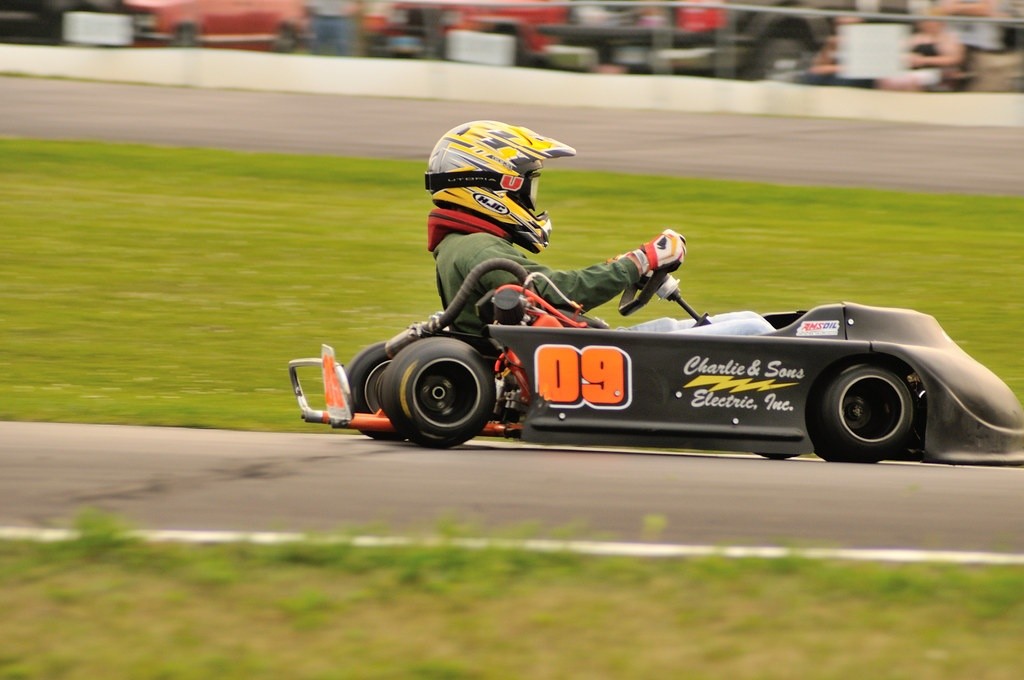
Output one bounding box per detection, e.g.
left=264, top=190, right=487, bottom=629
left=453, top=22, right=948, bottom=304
left=428, top=119, right=688, bottom=336
left=802, top=0, right=1018, bottom=93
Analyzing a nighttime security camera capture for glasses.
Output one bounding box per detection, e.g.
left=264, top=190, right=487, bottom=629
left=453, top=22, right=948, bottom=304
left=505, top=170, right=541, bottom=211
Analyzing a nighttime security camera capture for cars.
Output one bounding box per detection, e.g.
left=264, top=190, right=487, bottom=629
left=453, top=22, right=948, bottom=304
left=2, top=0, right=980, bottom=89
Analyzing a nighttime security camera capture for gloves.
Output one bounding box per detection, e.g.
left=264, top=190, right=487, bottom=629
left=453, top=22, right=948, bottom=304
left=619, top=229, right=687, bottom=283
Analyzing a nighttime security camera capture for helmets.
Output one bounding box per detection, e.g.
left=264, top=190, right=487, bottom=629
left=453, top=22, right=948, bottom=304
left=425, top=120, right=577, bottom=254
